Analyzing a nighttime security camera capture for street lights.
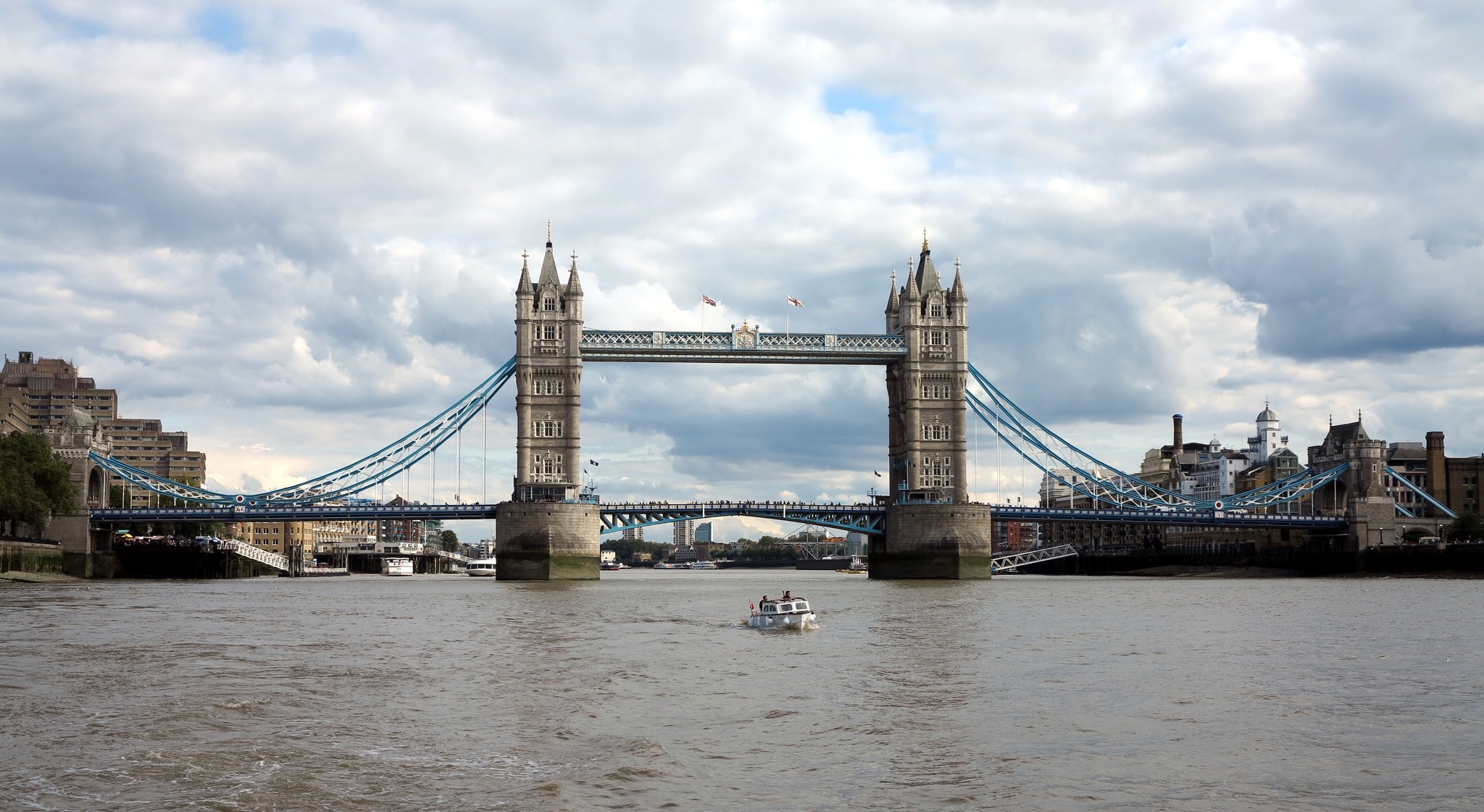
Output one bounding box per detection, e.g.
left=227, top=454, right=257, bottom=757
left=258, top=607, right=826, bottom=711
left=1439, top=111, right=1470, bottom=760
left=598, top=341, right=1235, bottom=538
left=1402, top=525, right=1406, bottom=542
left=1439, top=523, right=1444, bottom=543
left=1378, top=527, right=1384, bottom=545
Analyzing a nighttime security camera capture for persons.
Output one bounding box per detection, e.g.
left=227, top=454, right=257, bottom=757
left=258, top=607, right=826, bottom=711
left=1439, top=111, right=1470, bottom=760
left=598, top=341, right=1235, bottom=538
left=1420, top=539, right=1481, bottom=544
left=988, top=502, right=1044, bottom=508
left=774, top=501, right=805, bottom=504
left=759, top=596, right=769, bottom=612
left=739, top=500, right=770, bottom=504
left=688, top=500, right=730, bottom=504
left=1314, top=513, right=1342, bottom=517
left=971, top=501, right=982, bottom=504
left=810, top=501, right=840, bottom=505
left=603, top=500, right=668, bottom=504
left=854, top=502, right=895, bottom=505
left=783, top=591, right=792, bottom=600
left=500, top=498, right=562, bottom=503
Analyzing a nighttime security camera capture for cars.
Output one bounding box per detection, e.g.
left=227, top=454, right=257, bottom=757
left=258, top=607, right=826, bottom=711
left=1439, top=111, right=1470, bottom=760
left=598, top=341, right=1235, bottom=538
left=828, top=554, right=839, bottom=557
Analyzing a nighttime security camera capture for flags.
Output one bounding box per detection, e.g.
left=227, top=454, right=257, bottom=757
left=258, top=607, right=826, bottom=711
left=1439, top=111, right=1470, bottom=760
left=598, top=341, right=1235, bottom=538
left=787, top=294, right=804, bottom=307
left=584, top=468, right=590, bottom=476
left=703, top=294, right=717, bottom=307
left=875, top=470, right=881, bottom=477
left=590, top=459, right=599, bottom=466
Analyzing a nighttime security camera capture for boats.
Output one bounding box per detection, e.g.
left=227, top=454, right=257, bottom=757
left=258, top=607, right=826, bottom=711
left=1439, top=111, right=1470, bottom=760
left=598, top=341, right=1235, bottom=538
left=747, top=595, right=817, bottom=632
left=464, top=557, right=496, bottom=577
left=652, top=561, right=719, bottom=570
left=381, top=557, right=414, bottom=576
left=600, top=560, right=631, bottom=570
left=835, top=545, right=869, bottom=574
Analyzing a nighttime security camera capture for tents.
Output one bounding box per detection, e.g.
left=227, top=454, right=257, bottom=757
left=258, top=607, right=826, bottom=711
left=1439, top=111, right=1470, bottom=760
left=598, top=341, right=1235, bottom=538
left=120, top=534, right=187, bottom=541
